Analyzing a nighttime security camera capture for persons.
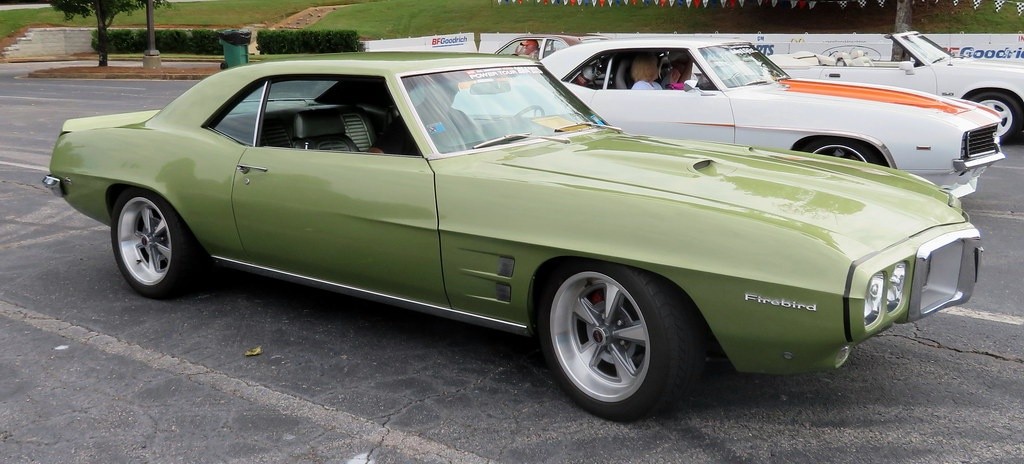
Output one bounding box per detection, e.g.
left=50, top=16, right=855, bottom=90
left=518, top=39, right=541, bottom=59
left=360, top=72, right=487, bottom=155
left=631, top=51, right=662, bottom=90
left=662, top=48, right=694, bottom=89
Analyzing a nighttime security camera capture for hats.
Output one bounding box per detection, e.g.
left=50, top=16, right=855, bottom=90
left=520, top=40, right=539, bottom=47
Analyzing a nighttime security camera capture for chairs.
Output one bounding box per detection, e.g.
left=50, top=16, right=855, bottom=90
left=836, top=51, right=875, bottom=66
left=262, top=105, right=378, bottom=152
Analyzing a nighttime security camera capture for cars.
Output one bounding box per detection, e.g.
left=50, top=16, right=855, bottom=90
left=43, top=54, right=985, bottom=423
left=773, top=30, right=1024, bottom=146
left=494, top=33, right=609, bottom=62
left=539, top=39, right=1005, bottom=206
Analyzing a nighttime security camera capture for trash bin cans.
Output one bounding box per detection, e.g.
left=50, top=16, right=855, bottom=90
left=216, top=29, right=252, bottom=70
